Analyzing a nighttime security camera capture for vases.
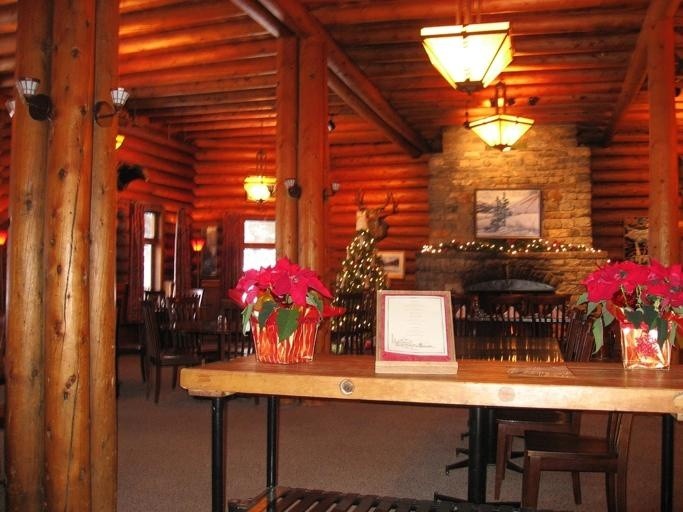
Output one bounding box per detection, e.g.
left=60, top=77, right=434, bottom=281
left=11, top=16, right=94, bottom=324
left=250, top=307, right=319, bottom=365
left=620, top=319, right=671, bottom=372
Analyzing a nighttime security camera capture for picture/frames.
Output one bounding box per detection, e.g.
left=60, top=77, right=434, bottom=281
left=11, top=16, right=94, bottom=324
left=474, top=188, right=541, bottom=240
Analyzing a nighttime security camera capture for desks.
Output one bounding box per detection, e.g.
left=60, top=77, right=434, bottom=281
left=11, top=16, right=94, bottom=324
left=179, top=353, right=683, bottom=512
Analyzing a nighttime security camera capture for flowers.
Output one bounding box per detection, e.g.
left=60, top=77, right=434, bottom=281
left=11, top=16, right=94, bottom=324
left=580, top=259, right=683, bottom=355
left=225, top=256, right=336, bottom=343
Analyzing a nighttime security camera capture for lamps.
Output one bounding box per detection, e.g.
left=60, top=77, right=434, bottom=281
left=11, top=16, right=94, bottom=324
left=94, top=88, right=131, bottom=128
left=116, top=135, right=126, bottom=150
left=243, top=121, right=277, bottom=208
left=4, top=98, right=15, bottom=118
left=282, top=177, right=301, bottom=199
left=420, top=0, right=514, bottom=96
left=15, top=75, right=54, bottom=121
left=191, top=238, right=205, bottom=287
left=462, top=80, right=536, bottom=153
left=323, top=180, right=341, bottom=199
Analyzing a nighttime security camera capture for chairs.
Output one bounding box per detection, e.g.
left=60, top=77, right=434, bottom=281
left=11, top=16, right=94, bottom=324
left=116, top=280, right=259, bottom=404
left=333, top=291, right=368, bottom=353
